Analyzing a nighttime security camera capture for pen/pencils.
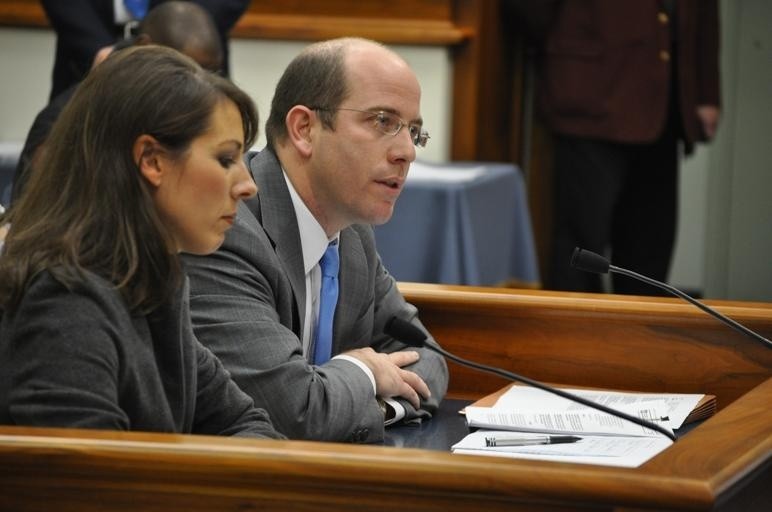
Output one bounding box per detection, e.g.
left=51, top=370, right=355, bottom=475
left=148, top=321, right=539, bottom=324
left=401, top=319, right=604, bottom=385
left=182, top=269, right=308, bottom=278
left=484, top=434, right=584, bottom=448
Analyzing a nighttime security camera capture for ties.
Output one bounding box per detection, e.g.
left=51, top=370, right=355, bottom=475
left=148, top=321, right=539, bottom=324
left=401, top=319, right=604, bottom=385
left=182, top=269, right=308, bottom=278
left=312, top=242, right=340, bottom=365
left=125, top=0, right=149, bottom=16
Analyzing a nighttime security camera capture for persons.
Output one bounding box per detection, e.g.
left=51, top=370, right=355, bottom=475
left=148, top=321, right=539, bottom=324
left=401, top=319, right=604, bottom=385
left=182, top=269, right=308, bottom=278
left=1, top=41, right=294, bottom=442
left=37, top=0, right=252, bottom=104
left=11, top=0, right=225, bottom=204
left=183, top=35, right=450, bottom=443
left=494, top=0, right=723, bottom=295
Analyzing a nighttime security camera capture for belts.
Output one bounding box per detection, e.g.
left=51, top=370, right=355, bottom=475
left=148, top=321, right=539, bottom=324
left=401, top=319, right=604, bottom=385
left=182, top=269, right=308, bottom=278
left=113, top=22, right=148, bottom=40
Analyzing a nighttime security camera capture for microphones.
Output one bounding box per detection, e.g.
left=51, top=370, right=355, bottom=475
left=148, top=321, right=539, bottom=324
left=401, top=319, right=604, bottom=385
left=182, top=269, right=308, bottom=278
left=569, top=245, right=772, bottom=350
left=382, top=313, right=677, bottom=442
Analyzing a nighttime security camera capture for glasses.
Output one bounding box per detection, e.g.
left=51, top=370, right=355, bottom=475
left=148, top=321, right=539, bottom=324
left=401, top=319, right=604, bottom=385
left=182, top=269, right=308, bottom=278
left=315, top=107, right=431, bottom=151
